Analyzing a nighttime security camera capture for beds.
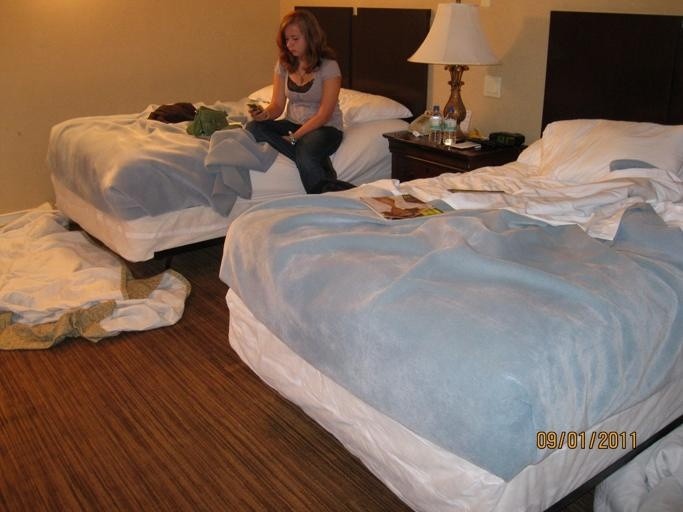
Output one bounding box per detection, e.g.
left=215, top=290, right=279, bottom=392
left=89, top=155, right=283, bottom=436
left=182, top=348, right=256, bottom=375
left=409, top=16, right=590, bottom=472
left=218, top=117, right=683, bottom=511
left=44, top=81, right=415, bottom=266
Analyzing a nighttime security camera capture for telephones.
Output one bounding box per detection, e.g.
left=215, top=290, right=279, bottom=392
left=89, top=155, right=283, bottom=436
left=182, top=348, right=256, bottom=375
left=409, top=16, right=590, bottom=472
left=408, top=110, right=442, bottom=137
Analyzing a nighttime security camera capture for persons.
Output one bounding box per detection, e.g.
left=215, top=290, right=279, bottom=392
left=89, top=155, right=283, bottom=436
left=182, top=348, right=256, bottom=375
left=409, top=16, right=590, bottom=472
left=246, top=9, right=356, bottom=194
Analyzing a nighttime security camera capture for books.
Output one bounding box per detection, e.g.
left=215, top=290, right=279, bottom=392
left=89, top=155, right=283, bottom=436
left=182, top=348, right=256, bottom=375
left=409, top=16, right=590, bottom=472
left=359, top=193, right=444, bottom=221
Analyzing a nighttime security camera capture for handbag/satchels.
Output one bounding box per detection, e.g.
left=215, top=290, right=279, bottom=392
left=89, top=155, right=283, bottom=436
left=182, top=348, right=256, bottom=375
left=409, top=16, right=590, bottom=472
left=187, top=106, right=228, bottom=137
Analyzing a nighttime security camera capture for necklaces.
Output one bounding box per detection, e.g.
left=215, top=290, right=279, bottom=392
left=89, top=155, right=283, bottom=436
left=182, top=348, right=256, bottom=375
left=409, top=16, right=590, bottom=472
left=296, top=70, right=309, bottom=84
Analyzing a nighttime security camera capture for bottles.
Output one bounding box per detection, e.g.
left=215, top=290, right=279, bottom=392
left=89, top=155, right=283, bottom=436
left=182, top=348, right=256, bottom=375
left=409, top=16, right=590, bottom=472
left=443, top=107, right=457, bottom=147
left=429, top=105, right=442, bottom=145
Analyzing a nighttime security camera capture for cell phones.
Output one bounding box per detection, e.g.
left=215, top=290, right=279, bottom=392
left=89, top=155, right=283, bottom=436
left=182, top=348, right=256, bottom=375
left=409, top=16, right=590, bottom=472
left=246, top=103, right=262, bottom=115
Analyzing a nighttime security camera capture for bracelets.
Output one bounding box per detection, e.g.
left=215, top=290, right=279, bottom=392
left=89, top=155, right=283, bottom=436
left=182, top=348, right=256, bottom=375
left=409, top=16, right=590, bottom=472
left=289, top=134, right=298, bottom=144
